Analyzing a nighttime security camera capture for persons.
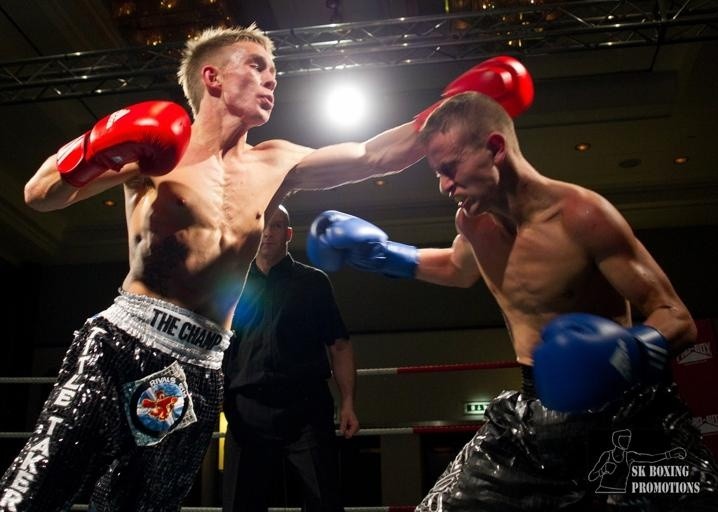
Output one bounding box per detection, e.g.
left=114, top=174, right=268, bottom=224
left=304, top=86, right=718, bottom=510
left=215, top=201, right=362, bottom=511
left=0, top=21, right=535, bottom=512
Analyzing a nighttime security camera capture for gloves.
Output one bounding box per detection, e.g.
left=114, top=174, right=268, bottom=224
left=305, top=209, right=418, bottom=280
left=413, top=55, right=535, bottom=133
left=531, top=312, right=674, bottom=413
left=56, top=100, right=192, bottom=188
